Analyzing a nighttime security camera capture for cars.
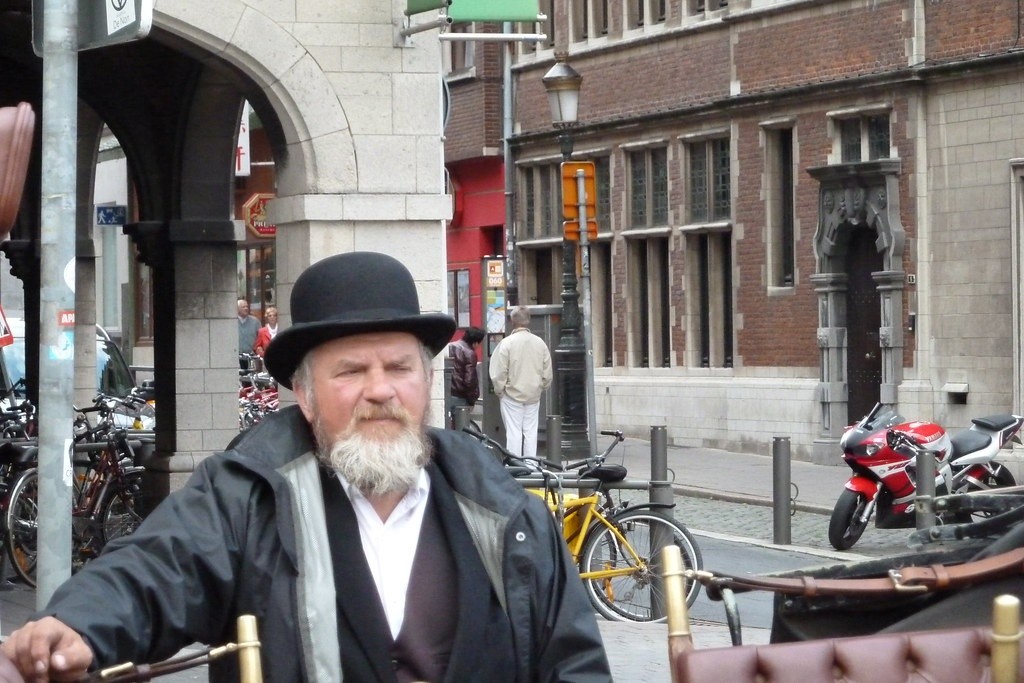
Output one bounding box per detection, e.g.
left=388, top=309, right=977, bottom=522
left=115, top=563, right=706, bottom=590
left=0, top=316, right=155, bottom=432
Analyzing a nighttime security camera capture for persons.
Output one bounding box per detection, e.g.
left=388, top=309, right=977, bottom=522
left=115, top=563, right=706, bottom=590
left=237, top=299, right=261, bottom=368
left=488, top=308, right=553, bottom=470
left=3, top=252, right=614, bottom=683
left=448, top=326, right=485, bottom=432
left=253, top=307, right=278, bottom=356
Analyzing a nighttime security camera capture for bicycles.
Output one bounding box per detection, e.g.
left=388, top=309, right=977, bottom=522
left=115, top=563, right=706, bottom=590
left=0, top=353, right=279, bottom=589
left=462, top=418, right=702, bottom=623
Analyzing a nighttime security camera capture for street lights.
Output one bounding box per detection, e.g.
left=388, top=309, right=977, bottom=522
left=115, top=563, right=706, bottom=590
left=543, top=53, right=593, bottom=468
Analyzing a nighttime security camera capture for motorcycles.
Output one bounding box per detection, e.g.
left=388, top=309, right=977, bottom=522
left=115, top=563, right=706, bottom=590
left=828, top=402, right=1024, bottom=551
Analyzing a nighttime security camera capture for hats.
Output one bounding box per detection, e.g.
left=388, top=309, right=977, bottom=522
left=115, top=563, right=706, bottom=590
left=264, top=252, right=457, bottom=390
